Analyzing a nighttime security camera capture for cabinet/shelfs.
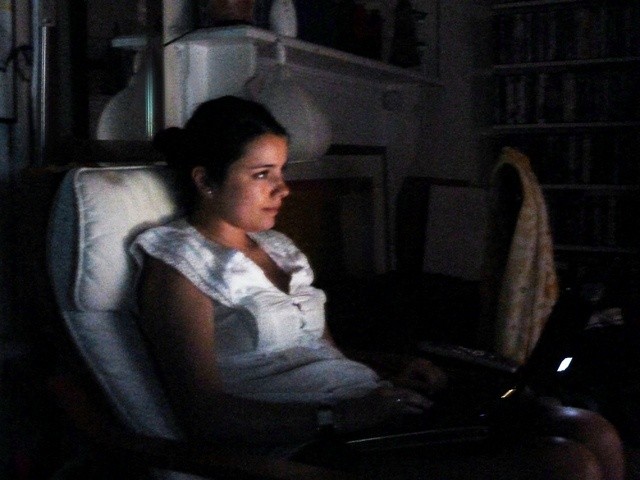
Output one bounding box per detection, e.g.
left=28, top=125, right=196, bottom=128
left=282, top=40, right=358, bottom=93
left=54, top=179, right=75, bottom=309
left=481, top=4, right=640, bottom=288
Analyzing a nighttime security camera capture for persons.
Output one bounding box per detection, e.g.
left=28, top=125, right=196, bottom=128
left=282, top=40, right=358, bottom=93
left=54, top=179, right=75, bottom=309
left=129, top=95, right=624, bottom=480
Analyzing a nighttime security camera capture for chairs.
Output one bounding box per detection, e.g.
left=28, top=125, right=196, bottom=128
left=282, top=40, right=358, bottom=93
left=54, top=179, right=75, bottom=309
left=48, top=165, right=534, bottom=478
